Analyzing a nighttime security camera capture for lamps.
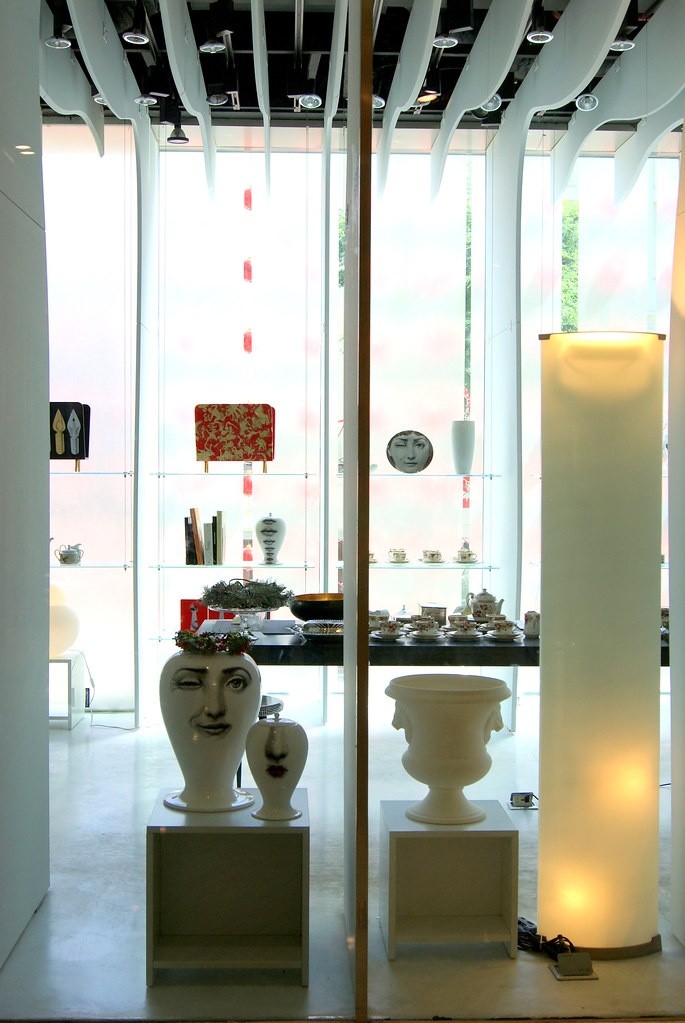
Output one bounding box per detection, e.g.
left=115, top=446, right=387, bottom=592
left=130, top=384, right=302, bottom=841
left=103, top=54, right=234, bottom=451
left=123, top=4, right=149, bottom=44
left=133, top=68, right=158, bottom=106
left=94, top=95, right=106, bottom=107
left=45, top=12, right=71, bottom=49
left=298, top=78, right=322, bottom=109
left=610, top=23, right=636, bottom=51
left=199, top=10, right=226, bottom=54
left=416, top=64, right=441, bottom=102
left=432, top=9, right=458, bottom=48
left=167, top=107, right=189, bottom=144
left=372, top=80, right=386, bottom=108
left=575, top=88, right=599, bottom=112
left=205, top=84, right=229, bottom=105
left=526, top=5, right=555, bottom=43
left=472, top=90, right=503, bottom=119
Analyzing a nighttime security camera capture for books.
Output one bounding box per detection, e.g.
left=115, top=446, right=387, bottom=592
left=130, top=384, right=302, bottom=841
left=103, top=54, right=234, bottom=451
left=185, top=508, right=224, bottom=566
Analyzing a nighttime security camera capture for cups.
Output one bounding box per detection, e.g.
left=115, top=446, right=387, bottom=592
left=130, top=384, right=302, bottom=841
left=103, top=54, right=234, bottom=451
left=457, top=549, right=477, bottom=561
left=387, top=547, right=406, bottom=561
left=369, top=613, right=514, bottom=636
left=369, top=553, right=374, bottom=560
left=422, top=550, right=443, bottom=561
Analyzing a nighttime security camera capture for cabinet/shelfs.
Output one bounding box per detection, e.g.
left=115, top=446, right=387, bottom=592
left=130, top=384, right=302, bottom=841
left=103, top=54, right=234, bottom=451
left=144, top=787, right=311, bottom=985
left=381, top=799, right=520, bottom=961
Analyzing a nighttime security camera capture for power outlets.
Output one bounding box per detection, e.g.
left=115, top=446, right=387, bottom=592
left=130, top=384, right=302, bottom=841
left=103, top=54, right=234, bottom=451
left=511, top=793, right=533, bottom=807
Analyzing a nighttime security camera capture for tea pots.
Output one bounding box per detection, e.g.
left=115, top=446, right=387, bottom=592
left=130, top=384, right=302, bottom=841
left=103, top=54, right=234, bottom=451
left=466, top=588, right=504, bottom=623
left=524, top=611, right=540, bottom=639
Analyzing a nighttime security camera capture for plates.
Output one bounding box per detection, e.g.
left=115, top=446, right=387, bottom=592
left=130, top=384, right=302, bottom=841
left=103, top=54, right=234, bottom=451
left=422, top=560, right=444, bottom=562
left=369, top=560, right=377, bottom=563
left=388, top=561, right=410, bottom=562
left=456, top=560, right=478, bottom=563
left=368, top=627, right=520, bottom=641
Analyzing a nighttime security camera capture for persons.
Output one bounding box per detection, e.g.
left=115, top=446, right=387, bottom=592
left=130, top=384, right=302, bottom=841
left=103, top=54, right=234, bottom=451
left=388, top=431, right=431, bottom=473
